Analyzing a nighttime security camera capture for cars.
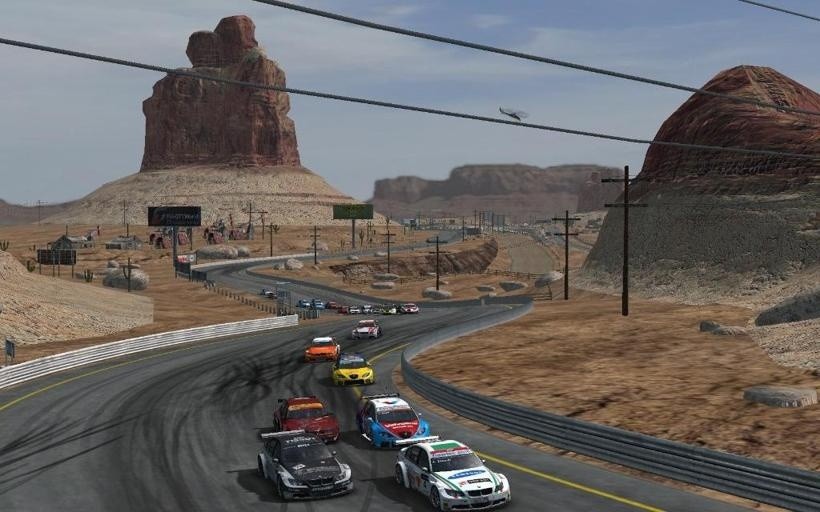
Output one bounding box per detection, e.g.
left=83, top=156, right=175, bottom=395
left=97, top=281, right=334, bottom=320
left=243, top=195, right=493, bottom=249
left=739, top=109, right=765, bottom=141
left=400, top=304, right=419, bottom=314
left=311, top=297, right=379, bottom=315
left=352, top=320, right=382, bottom=340
left=296, top=299, right=310, bottom=307
left=258, top=430, right=353, bottom=500
left=332, top=352, right=374, bottom=386
left=357, top=393, right=431, bottom=450
left=395, top=436, right=511, bottom=512
left=380, top=304, right=400, bottom=314
left=304, top=336, right=340, bottom=362
left=273, top=396, right=339, bottom=444
